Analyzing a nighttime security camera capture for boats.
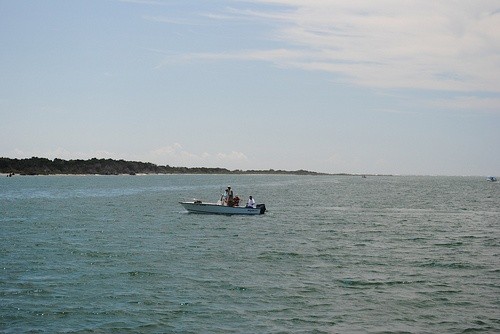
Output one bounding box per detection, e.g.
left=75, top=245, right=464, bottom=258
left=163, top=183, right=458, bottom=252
left=178, top=202, right=260, bottom=215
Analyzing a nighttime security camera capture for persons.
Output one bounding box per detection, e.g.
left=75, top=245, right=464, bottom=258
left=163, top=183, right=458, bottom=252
left=245, top=196, right=255, bottom=208
left=221, top=185, right=240, bottom=206
